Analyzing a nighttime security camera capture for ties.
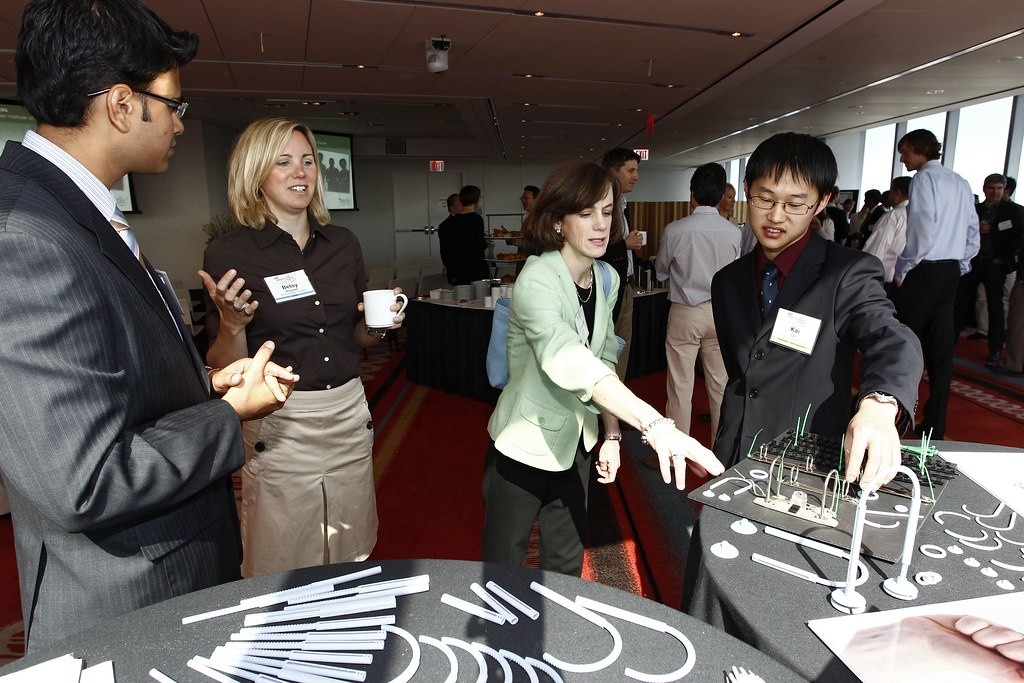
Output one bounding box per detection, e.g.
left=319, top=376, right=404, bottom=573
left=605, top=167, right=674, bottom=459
left=111, top=206, right=138, bottom=256
left=758, top=263, right=781, bottom=320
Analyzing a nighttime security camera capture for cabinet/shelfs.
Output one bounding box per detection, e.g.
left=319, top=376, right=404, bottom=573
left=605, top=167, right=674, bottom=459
left=484, top=213, right=527, bottom=288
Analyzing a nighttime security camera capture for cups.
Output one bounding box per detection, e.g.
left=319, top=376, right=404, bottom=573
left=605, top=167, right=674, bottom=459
left=363, top=289, right=408, bottom=329
left=636, top=231, right=647, bottom=246
left=484, top=284, right=513, bottom=308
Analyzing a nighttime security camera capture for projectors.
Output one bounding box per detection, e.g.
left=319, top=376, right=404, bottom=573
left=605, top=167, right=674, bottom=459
left=425, top=48, right=449, bottom=73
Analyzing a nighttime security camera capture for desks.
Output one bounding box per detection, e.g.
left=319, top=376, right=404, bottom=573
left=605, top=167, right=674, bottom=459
left=0, top=558, right=812, bottom=683
left=406, top=288, right=674, bottom=407
left=681, top=440, right=1024, bottom=683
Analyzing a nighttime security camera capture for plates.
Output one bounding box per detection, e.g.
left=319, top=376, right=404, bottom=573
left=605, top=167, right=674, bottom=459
left=442, top=279, right=491, bottom=305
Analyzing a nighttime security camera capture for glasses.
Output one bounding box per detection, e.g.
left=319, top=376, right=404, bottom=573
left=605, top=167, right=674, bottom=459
left=88, top=87, right=189, bottom=120
left=749, top=196, right=820, bottom=215
left=520, top=197, right=531, bottom=201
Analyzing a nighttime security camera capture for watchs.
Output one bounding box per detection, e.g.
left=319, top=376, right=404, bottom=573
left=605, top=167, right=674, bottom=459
left=859, top=391, right=899, bottom=416
left=604, top=433, right=622, bottom=441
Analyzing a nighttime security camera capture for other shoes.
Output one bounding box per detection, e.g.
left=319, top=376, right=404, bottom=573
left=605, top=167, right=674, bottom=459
left=984, top=353, right=1000, bottom=369
left=912, top=423, right=927, bottom=438
left=967, top=331, right=986, bottom=339
left=994, top=365, right=1022, bottom=377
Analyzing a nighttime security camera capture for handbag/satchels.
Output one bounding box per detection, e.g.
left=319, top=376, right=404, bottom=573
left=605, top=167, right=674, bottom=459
left=486, top=261, right=625, bottom=390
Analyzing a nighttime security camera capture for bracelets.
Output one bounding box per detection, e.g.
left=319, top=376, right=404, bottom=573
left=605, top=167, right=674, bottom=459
left=366, top=326, right=386, bottom=339
left=641, top=418, right=676, bottom=444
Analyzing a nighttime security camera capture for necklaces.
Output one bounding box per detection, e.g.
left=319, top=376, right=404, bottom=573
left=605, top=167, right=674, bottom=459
left=573, top=265, right=593, bottom=302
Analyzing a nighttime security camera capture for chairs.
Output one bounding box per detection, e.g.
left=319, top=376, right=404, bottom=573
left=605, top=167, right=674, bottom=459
left=174, top=256, right=518, bottom=361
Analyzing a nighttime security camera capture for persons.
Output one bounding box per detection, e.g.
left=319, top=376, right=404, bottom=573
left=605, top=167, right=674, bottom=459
left=494, top=185, right=541, bottom=276
left=718, top=182, right=739, bottom=227
left=199, top=118, right=405, bottom=579
left=318, top=152, right=326, bottom=183
left=642, top=161, right=742, bottom=467
left=451, top=185, right=490, bottom=285
left=740, top=178, right=757, bottom=255
left=602, top=147, right=640, bottom=382
left=339, top=158, right=350, bottom=192
left=482, top=161, right=727, bottom=579
left=327, top=158, right=340, bottom=192
left=0, top=0, right=299, bottom=658
left=438, top=194, right=462, bottom=285
left=893, top=128, right=981, bottom=439
left=683, top=132, right=926, bottom=492
left=811, top=173, right=1024, bottom=377
